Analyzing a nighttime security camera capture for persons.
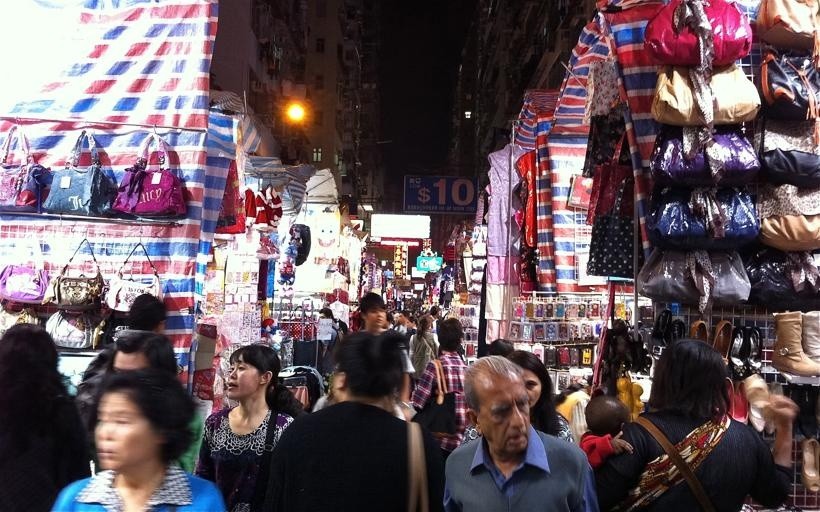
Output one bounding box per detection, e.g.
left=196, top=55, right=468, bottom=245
left=0, top=293, right=801, bottom=512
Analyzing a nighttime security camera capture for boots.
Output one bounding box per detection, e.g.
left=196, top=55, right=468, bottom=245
left=772, top=311, right=819, bottom=376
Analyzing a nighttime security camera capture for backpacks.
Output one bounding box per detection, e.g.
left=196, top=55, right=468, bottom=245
left=73, top=342, right=116, bottom=428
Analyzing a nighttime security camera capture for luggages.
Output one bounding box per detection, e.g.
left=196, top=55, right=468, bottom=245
left=273, top=296, right=320, bottom=409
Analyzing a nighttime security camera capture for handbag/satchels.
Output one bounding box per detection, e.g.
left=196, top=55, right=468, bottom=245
left=643, top=0, right=752, bottom=80
left=646, top=182, right=758, bottom=242
left=0, top=235, right=163, bottom=350
left=753, top=108, right=820, bottom=180
left=755, top=175, right=820, bottom=251
left=468, top=231, right=486, bottom=305
left=649, top=123, right=761, bottom=182
left=423, top=362, right=457, bottom=438
left=0, top=126, right=187, bottom=217
left=755, top=44, right=820, bottom=118
left=746, top=247, right=820, bottom=309
left=755, top=0, right=820, bottom=62
left=637, top=245, right=752, bottom=313
left=650, top=63, right=762, bottom=124
left=581, top=63, right=642, bottom=277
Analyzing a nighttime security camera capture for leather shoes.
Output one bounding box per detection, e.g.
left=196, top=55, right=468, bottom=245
left=801, top=440, right=820, bottom=493
left=650, top=309, right=764, bottom=379
left=728, top=377, right=820, bottom=439
